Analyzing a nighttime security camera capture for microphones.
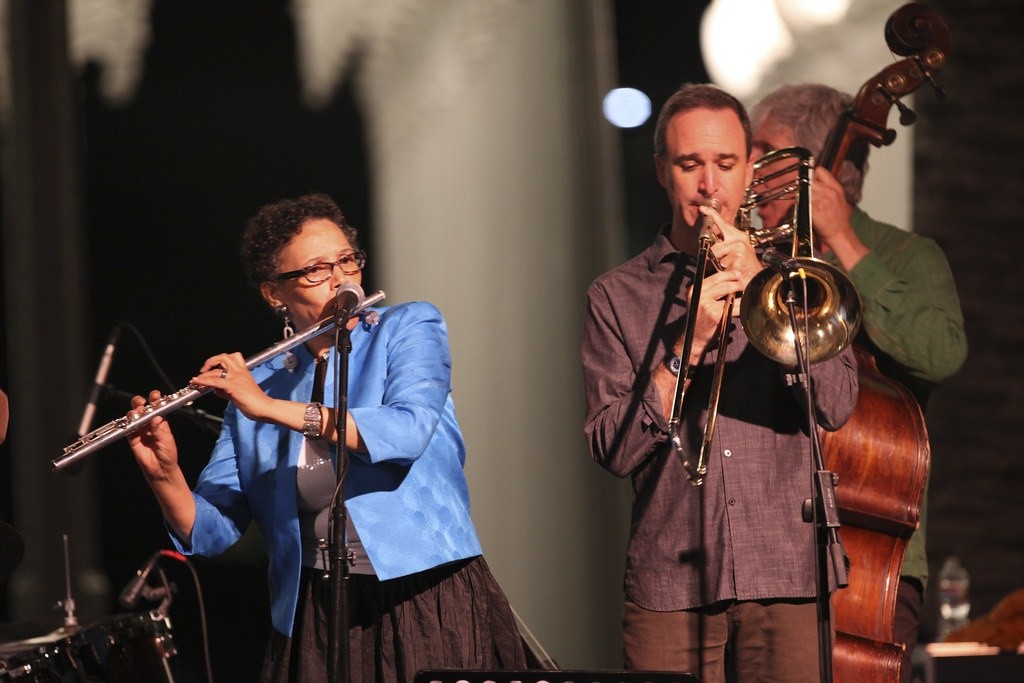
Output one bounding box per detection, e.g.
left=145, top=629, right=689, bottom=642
left=335, top=283, right=364, bottom=328
left=120, top=550, right=164, bottom=608
left=762, top=250, right=803, bottom=272
left=79, top=328, right=120, bottom=437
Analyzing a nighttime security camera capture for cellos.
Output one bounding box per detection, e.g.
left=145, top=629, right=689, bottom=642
left=784, top=2, right=956, bottom=682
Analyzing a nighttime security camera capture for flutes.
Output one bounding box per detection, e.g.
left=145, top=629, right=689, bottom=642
left=48, top=290, right=389, bottom=471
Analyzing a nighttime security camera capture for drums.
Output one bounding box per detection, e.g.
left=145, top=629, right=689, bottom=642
left=32, top=620, right=169, bottom=683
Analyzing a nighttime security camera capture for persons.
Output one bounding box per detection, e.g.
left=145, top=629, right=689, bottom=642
left=128, top=194, right=525, bottom=683
left=749, top=86, right=968, bottom=683
left=584, top=84, right=858, bottom=683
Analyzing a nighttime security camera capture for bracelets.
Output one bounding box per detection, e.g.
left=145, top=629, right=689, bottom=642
left=302, top=402, right=323, bottom=439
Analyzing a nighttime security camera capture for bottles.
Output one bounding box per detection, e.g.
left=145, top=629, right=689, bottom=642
left=938, top=556, right=970, bottom=641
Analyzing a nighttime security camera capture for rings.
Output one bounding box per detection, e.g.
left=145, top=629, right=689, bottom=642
left=717, top=256, right=728, bottom=270
left=220, top=369, right=228, bottom=378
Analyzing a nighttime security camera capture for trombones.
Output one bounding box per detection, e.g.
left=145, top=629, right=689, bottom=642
left=669, top=146, right=864, bottom=490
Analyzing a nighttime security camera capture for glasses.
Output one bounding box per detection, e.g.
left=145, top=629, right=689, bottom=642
left=276, top=251, right=365, bottom=283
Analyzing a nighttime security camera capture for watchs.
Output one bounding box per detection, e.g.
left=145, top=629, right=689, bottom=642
left=663, top=346, right=701, bottom=381
